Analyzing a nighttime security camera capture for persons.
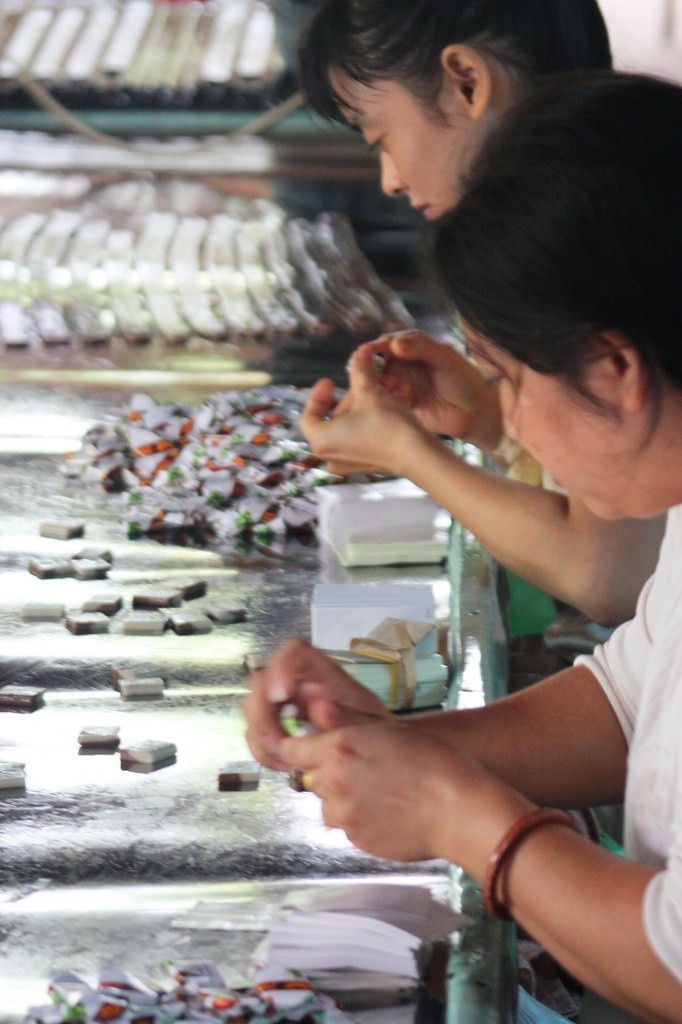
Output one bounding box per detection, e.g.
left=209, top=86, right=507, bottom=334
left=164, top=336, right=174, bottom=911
left=294, top=0, right=669, bottom=627
left=239, top=70, right=682, bottom=1024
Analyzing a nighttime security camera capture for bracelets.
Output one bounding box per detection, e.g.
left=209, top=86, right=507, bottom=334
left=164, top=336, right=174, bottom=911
left=483, top=806, right=587, bottom=922
